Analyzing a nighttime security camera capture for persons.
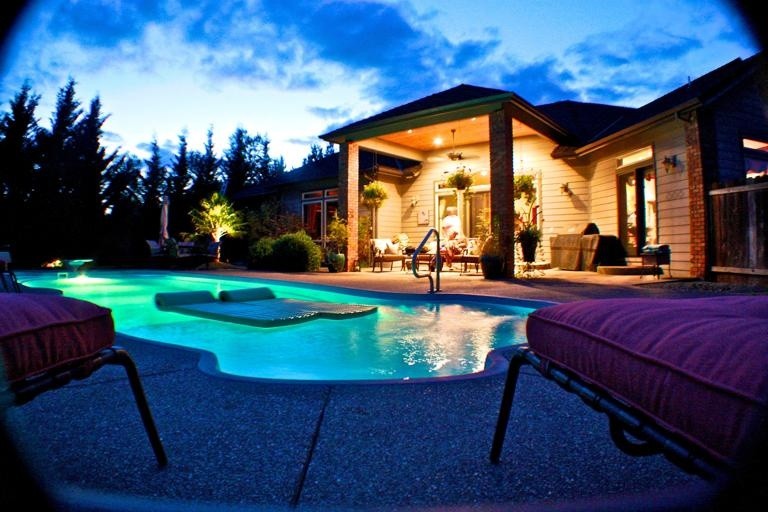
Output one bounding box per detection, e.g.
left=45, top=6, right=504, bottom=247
left=405, top=204, right=469, bottom=268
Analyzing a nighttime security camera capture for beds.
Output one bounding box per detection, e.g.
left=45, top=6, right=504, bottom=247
left=154, top=287, right=378, bottom=328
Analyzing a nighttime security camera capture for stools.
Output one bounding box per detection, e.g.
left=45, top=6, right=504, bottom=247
left=489, top=296, right=767, bottom=511
left=0, top=289, right=171, bottom=475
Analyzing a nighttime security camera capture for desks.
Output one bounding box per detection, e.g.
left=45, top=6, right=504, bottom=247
left=639, top=253, right=673, bottom=279
left=550, top=235, right=616, bottom=271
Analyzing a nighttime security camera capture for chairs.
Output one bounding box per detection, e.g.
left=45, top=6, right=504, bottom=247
left=145, top=237, right=222, bottom=271
left=368, top=237, right=480, bottom=273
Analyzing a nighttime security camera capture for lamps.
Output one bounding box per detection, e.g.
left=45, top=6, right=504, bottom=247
left=661, top=155, right=677, bottom=174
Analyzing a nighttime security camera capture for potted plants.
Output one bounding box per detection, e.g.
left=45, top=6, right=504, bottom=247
left=480, top=197, right=545, bottom=280
left=363, top=180, right=388, bottom=209
left=323, top=218, right=353, bottom=274
left=448, top=168, right=473, bottom=190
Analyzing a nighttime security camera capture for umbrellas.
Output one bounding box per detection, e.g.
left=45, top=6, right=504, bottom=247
left=159, top=195, right=169, bottom=246
